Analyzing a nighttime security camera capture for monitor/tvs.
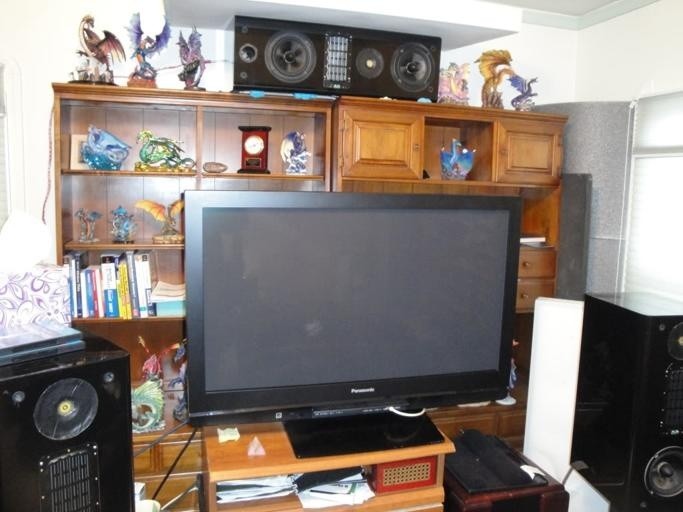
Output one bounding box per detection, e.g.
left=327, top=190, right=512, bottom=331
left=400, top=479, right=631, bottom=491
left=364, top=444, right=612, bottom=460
left=182, top=191, right=521, bottom=458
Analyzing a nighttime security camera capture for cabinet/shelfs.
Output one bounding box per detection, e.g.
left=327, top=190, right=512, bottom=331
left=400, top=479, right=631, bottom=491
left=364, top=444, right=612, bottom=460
left=49, top=80, right=337, bottom=326
left=128, top=365, right=527, bottom=511
left=333, top=96, right=568, bottom=315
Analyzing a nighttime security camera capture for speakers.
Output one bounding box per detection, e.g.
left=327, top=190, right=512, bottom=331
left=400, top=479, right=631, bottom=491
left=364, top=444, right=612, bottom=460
left=568, top=290, right=683, bottom=511
left=233, top=13, right=442, bottom=103
left=0, top=336, right=136, bottom=512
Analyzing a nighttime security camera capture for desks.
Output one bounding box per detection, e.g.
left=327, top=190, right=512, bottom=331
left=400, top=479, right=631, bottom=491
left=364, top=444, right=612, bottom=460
left=443, top=445, right=570, bottom=510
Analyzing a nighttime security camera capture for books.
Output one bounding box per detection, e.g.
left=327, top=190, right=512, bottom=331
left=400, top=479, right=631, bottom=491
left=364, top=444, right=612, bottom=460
left=63, top=251, right=187, bottom=320
left=519, top=234, right=546, bottom=243
